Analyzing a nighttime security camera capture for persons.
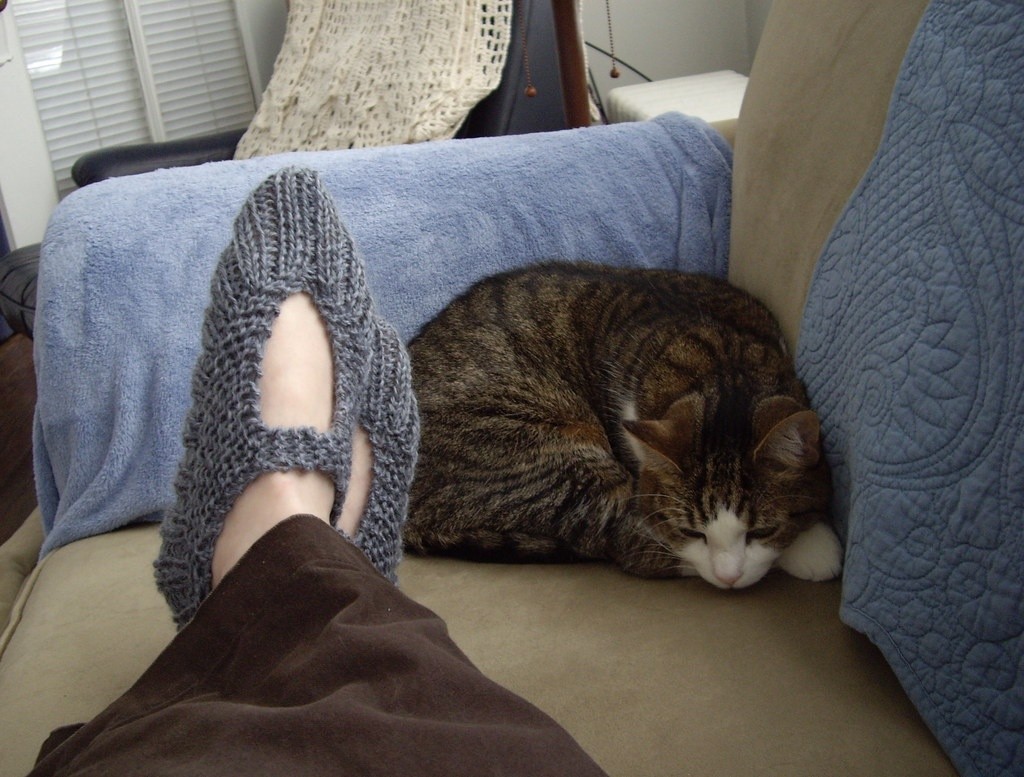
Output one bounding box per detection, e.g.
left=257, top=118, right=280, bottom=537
left=38, top=167, right=613, bottom=777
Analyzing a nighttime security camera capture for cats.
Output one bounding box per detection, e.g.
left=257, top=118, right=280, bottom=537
left=402, top=260, right=842, bottom=590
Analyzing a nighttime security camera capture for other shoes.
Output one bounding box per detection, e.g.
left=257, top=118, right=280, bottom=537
left=154, top=164, right=376, bottom=633
left=352, top=314, right=419, bottom=589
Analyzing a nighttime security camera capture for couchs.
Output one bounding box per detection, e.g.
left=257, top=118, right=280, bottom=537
left=0, top=1, right=1024, bottom=774
